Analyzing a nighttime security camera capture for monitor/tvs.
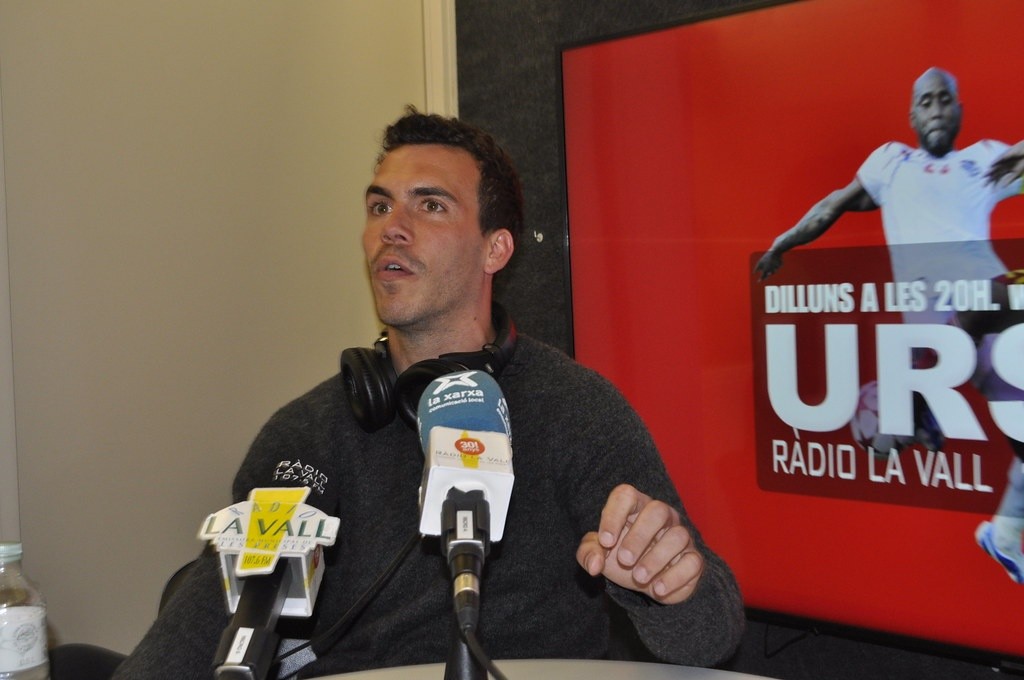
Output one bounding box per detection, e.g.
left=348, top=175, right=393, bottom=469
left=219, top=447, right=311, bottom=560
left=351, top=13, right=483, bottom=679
left=552, top=0, right=1024, bottom=680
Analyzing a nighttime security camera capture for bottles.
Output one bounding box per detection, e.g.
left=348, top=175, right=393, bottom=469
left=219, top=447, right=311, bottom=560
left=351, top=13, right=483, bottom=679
left=0, top=541, right=49, bottom=680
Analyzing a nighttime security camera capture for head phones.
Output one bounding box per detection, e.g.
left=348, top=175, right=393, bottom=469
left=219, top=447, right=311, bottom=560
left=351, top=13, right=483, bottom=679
left=340, top=302, right=517, bottom=434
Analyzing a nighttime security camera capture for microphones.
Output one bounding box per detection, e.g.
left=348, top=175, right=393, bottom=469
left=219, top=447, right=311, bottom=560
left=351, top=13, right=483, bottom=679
left=417, top=370, right=514, bottom=639
left=199, top=478, right=339, bottom=680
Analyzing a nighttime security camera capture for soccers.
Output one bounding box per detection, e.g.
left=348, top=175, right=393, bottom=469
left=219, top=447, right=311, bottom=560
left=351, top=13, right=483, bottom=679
left=849, top=380, right=911, bottom=459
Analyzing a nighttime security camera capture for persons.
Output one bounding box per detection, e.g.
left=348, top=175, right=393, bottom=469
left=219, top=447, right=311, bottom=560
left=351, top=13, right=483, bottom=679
left=755, top=67, right=1024, bottom=585
left=113, top=102, right=749, bottom=680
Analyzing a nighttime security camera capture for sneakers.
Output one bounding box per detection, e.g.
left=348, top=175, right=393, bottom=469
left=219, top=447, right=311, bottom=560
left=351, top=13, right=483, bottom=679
left=974, top=520, right=1024, bottom=585
